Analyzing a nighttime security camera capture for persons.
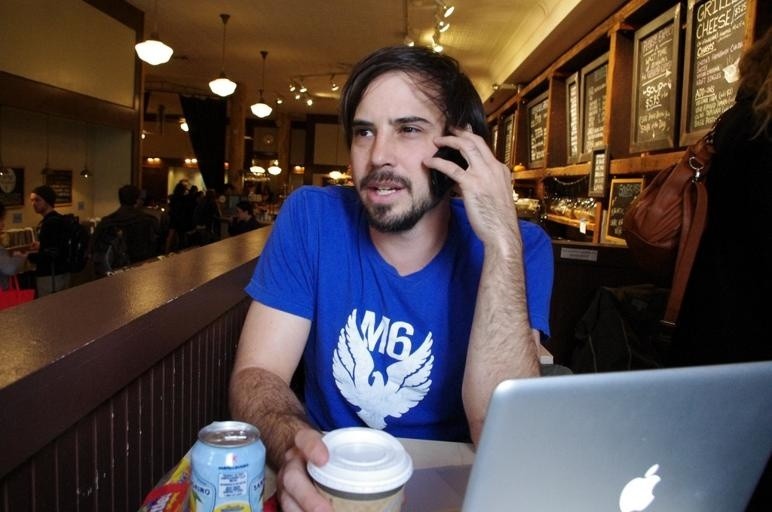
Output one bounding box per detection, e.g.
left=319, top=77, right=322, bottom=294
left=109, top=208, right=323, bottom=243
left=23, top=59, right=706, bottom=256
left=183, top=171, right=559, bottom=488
left=168, top=181, right=276, bottom=240
left=0, top=202, right=26, bottom=292
left=228, top=47, right=554, bottom=512
left=13, top=186, right=91, bottom=298
left=92, top=184, right=162, bottom=263
left=694, top=27, right=772, bottom=364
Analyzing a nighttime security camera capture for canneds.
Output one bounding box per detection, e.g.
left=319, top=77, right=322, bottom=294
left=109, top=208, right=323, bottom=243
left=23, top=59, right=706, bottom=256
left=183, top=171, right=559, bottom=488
left=189, top=419, right=267, bottom=512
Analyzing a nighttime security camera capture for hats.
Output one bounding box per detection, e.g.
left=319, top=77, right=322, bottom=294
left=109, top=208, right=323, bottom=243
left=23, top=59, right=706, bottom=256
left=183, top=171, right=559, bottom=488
left=30, top=184, right=55, bottom=208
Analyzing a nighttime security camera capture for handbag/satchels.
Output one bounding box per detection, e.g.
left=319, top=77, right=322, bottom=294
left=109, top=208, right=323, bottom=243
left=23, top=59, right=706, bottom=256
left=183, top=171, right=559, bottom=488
left=621, top=136, right=714, bottom=273
left=0, top=274, right=34, bottom=308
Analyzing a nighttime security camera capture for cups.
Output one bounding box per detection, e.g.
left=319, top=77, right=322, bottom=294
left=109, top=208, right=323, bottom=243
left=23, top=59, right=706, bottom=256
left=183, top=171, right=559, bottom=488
left=514, top=191, right=596, bottom=226
left=305, top=426, right=416, bottom=512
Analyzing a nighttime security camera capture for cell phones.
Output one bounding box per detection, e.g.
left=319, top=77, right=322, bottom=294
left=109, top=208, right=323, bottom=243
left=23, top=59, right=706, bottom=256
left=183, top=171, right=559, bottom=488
left=430, top=146, right=468, bottom=196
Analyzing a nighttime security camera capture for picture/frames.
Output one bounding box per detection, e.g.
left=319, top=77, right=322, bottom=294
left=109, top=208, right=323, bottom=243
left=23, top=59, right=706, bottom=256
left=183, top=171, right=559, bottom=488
left=0, top=167, right=24, bottom=208
left=45, top=170, right=72, bottom=206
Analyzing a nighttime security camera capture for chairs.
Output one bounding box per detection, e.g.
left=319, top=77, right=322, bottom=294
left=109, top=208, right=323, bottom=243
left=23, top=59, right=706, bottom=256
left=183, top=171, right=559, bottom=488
left=42, top=221, right=123, bottom=293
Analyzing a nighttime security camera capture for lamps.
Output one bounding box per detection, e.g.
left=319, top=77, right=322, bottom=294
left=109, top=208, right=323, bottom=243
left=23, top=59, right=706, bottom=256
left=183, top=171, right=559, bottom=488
left=41, top=111, right=56, bottom=175
left=328, top=79, right=339, bottom=92
left=135, top=0, right=174, bottom=67
left=207, top=13, right=238, bottom=97
left=267, top=166, right=281, bottom=176
left=81, top=133, right=93, bottom=177
left=250, top=166, right=265, bottom=175
left=432, top=1, right=455, bottom=54
left=276, top=78, right=314, bottom=108
left=250, top=50, right=273, bottom=117
left=329, top=171, right=342, bottom=180
left=403, top=36, right=416, bottom=49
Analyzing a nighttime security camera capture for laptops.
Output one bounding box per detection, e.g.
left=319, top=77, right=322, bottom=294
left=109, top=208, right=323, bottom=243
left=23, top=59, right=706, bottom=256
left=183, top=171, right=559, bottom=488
left=402, top=360, right=772, bottom=512
